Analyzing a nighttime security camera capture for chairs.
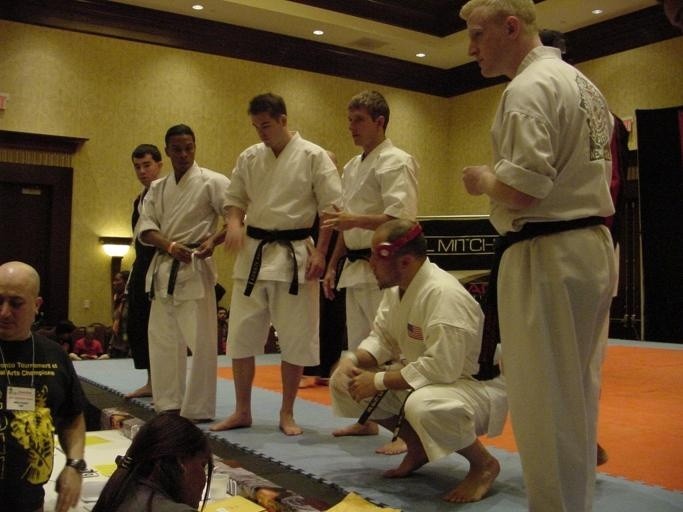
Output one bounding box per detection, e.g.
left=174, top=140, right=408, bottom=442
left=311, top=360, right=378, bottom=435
left=33, top=322, right=113, bottom=359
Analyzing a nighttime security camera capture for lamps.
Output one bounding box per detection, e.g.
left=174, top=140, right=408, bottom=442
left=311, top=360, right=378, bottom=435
left=98, top=237, right=132, bottom=259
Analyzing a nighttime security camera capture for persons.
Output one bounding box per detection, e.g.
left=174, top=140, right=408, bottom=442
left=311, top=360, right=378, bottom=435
left=460, top=1, right=618, bottom=511
left=208, top=93, right=343, bottom=436
left=214, top=304, right=227, bottom=355
left=44, top=319, right=76, bottom=354
left=533, top=28, right=629, bottom=299
left=133, top=124, right=239, bottom=424
left=320, top=90, right=420, bottom=455
left=108, top=269, right=130, bottom=359
left=89, top=413, right=215, bottom=511
left=0, top=259, right=88, bottom=511
left=124, top=144, right=163, bottom=400
left=328, top=219, right=509, bottom=503
left=67, top=326, right=109, bottom=360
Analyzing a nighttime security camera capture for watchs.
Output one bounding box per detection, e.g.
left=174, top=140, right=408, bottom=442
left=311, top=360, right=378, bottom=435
left=65, top=457, right=86, bottom=473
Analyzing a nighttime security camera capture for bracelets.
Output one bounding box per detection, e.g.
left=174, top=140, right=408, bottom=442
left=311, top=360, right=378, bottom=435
left=372, top=370, right=389, bottom=393
left=166, top=240, right=179, bottom=255
